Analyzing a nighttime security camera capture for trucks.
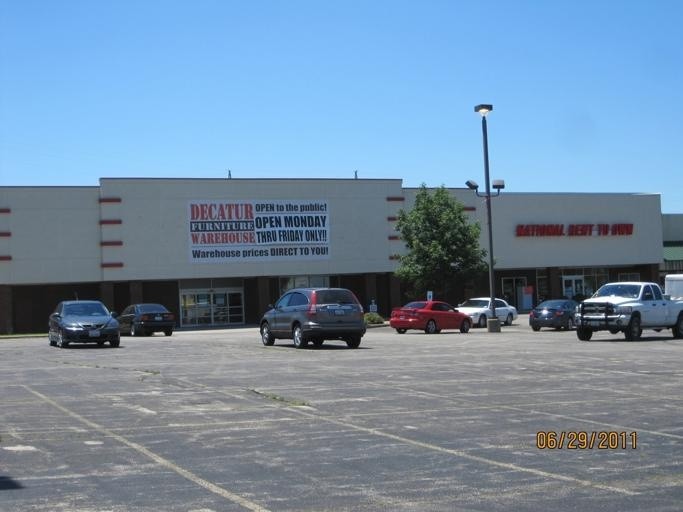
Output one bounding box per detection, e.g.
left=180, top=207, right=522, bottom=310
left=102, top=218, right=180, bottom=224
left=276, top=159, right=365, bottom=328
left=664, top=274, right=683, bottom=298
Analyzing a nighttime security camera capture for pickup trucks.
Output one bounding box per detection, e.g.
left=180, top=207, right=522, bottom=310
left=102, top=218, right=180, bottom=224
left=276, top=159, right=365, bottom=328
left=574, top=282, right=683, bottom=342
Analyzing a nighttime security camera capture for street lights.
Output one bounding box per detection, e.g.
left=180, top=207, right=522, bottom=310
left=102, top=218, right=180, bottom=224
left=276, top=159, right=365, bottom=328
left=465, top=102, right=507, bottom=331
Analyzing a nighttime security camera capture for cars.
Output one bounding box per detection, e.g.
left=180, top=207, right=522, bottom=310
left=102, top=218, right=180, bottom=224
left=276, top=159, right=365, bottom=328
left=449, top=297, right=518, bottom=328
left=48, top=300, right=121, bottom=346
left=391, top=301, right=473, bottom=334
left=116, top=303, right=176, bottom=337
left=528, top=299, right=577, bottom=331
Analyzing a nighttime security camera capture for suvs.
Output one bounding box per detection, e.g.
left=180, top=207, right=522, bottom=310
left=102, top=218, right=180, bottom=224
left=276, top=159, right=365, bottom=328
left=261, top=287, right=366, bottom=349
